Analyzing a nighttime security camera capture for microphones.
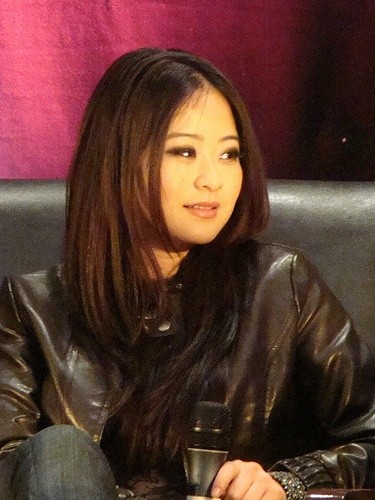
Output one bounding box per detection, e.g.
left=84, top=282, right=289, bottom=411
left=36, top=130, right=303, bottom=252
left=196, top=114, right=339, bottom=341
left=183, top=403, right=232, bottom=499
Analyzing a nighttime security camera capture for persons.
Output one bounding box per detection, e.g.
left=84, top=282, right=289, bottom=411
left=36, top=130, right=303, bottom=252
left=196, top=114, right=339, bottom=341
left=0, top=49, right=375, bottom=500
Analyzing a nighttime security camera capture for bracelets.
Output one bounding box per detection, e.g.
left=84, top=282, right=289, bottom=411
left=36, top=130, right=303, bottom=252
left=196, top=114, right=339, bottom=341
left=269, top=470, right=308, bottom=500
